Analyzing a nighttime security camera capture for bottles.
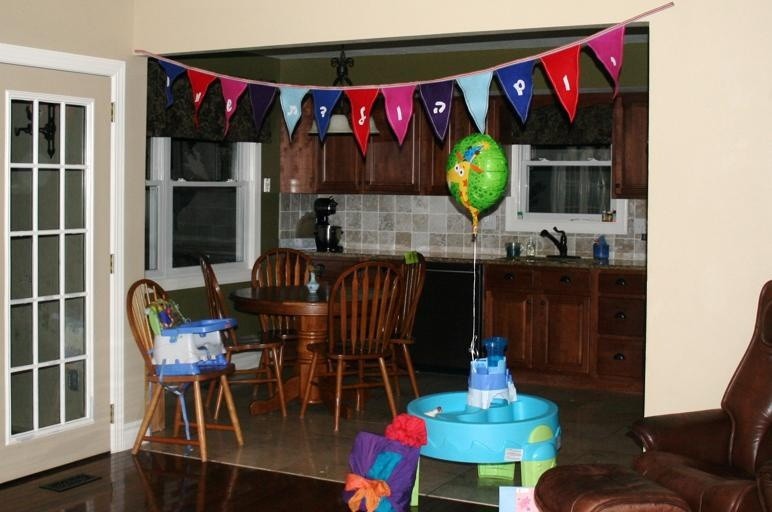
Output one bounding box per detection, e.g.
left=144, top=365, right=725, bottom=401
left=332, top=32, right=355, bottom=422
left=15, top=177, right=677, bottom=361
left=306, top=271, right=320, bottom=294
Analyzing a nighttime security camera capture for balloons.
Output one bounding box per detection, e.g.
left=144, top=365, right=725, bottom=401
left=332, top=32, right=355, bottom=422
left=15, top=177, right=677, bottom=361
left=446, top=134, right=509, bottom=241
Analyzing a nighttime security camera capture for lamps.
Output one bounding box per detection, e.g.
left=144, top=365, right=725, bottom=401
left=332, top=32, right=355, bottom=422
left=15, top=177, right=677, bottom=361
left=308, top=46, right=380, bottom=134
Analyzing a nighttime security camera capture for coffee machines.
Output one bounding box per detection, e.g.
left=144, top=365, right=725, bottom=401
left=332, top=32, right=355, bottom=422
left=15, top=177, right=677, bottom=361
left=313, top=195, right=345, bottom=254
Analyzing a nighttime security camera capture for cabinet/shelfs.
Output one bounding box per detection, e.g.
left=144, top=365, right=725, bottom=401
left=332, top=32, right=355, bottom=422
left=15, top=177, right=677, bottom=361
left=614, top=94, right=649, bottom=200
left=596, top=269, right=645, bottom=386
left=481, top=262, right=594, bottom=386
left=409, top=263, right=481, bottom=377
left=313, top=90, right=501, bottom=196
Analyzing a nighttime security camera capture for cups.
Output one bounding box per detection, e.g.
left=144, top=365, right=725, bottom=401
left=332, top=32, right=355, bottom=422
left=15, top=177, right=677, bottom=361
left=504, top=240, right=524, bottom=260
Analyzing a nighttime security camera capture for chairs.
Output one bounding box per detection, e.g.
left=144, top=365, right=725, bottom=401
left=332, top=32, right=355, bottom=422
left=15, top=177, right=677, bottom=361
left=127, top=247, right=427, bottom=433
left=535, top=280, right=772, bottom=512
left=129, top=279, right=244, bottom=459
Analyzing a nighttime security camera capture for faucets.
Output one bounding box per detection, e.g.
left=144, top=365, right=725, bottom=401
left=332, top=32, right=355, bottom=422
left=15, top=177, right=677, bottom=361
left=540, top=227, right=568, bottom=255
